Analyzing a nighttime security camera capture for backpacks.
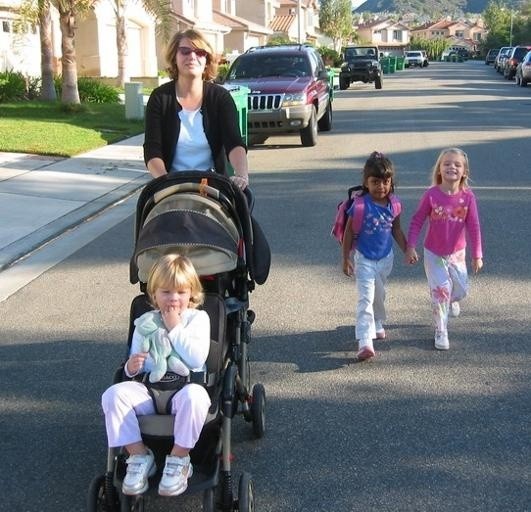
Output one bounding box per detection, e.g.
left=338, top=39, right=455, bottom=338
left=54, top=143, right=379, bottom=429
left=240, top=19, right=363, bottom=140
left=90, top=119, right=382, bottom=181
left=330, top=186, right=401, bottom=257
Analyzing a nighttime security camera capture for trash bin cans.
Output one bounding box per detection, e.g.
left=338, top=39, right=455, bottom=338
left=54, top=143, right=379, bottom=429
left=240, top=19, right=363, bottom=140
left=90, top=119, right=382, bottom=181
left=379, top=57, right=390, bottom=75
left=215, top=83, right=250, bottom=177
left=396, top=55, right=405, bottom=70
left=326, top=68, right=334, bottom=102
left=388, top=55, right=397, bottom=73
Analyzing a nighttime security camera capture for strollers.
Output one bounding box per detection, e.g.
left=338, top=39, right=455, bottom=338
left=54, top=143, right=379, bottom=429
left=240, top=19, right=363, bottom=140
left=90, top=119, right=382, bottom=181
left=85, top=171, right=273, bottom=512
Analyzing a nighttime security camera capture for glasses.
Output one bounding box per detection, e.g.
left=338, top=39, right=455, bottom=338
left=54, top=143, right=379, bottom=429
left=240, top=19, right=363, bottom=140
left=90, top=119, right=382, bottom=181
left=177, top=46, right=206, bottom=57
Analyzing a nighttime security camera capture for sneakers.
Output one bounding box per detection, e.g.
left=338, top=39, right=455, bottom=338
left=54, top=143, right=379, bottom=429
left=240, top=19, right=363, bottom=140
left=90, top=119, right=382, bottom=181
left=357, top=339, right=375, bottom=359
left=122, top=448, right=156, bottom=496
left=451, top=301, right=460, bottom=316
left=434, top=330, right=449, bottom=350
left=158, top=454, right=193, bottom=497
left=376, top=320, right=385, bottom=338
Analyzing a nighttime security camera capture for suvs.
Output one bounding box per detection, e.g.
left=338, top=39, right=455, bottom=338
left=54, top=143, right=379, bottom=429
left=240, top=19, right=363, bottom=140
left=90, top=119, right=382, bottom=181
left=220, top=43, right=334, bottom=146
left=339, top=47, right=383, bottom=90
left=404, top=51, right=427, bottom=68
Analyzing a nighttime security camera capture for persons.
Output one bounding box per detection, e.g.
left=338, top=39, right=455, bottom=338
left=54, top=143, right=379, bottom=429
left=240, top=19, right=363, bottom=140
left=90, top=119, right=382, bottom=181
left=342, top=151, right=418, bottom=361
left=406, top=148, right=483, bottom=350
left=101, top=253, right=211, bottom=497
left=142, top=29, right=249, bottom=192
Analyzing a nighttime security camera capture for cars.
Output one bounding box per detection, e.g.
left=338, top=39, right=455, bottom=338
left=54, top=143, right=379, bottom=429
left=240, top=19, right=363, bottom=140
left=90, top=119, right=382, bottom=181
left=485, top=46, right=531, bottom=86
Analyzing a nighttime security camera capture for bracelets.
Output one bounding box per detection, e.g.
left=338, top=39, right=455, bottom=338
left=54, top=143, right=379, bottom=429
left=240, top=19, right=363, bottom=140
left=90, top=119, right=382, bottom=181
left=237, top=175, right=248, bottom=180
